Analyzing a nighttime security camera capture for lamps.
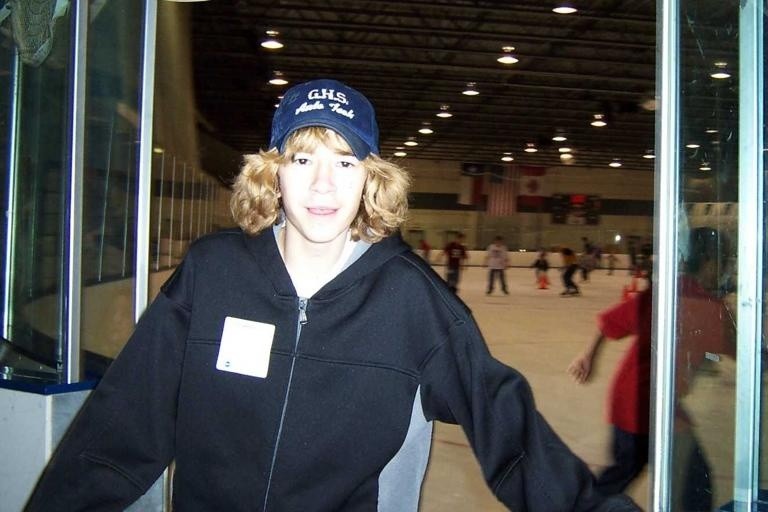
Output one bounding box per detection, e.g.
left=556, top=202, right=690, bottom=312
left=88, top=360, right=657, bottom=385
left=500, top=151, right=515, bottom=162
left=436, top=102, right=453, bottom=119
left=686, top=138, right=702, bottom=150
left=550, top=129, right=568, bottom=142
left=418, top=121, right=434, bottom=135
left=703, top=122, right=719, bottom=135
left=268, top=68, right=289, bottom=85
left=709, top=62, right=731, bottom=79
left=496, top=44, right=520, bottom=65
left=609, top=157, right=623, bottom=167
left=698, top=160, right=713, bottom=171
left=643, top=147, right=656, bottom=159
left=524, top=142, right=537, bottom=153
left=558, top=146, right=571, bottom=153
left=559, top=154, right=573, bottom=159
left=393, top=146, right=407, bottom=158
left=461, top=80, right=480, bottom=97
left=261, top=25, right=285, bottom=50
left=273, top=96, right=286, bottom=108
left=404, top=135, right=418, bottom=146
left=549, top=1, right=579, bottom=17
left=590, top=113, right=608, bottom=127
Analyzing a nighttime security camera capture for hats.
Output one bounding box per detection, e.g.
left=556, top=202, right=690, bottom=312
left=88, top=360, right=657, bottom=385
left=269, top=80, right=380, bottom=161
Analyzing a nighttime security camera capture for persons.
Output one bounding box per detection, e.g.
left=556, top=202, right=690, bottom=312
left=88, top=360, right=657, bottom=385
left=568, top=226, right=768, bottom=511
left=577, top=235, right=601, bottom=281
left=530, top=248, right=549, bottom=288
left=551, top=244, right=579, bottom=296
left=482, top=235, right=510, bottom=294
left=25, top=78, right=640, bottom=511
left=438, top=233, right=467, bottom=287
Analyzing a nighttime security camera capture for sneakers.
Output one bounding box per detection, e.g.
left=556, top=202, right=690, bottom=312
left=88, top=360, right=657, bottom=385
left=486, top=288, right=510, bottom=294
left=560, top=290, right=579, bottom=295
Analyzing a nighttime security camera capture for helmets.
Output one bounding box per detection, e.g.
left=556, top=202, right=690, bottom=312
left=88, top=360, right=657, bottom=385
left=686, top=226, right=728, bottom=266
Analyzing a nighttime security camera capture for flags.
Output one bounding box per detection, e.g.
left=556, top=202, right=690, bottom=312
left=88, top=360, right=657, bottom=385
left=456, top=161, right=484, bottom=206
left=517, top=165, right=545, bottom=210
left=486, top=164, right=517, bottom=217
